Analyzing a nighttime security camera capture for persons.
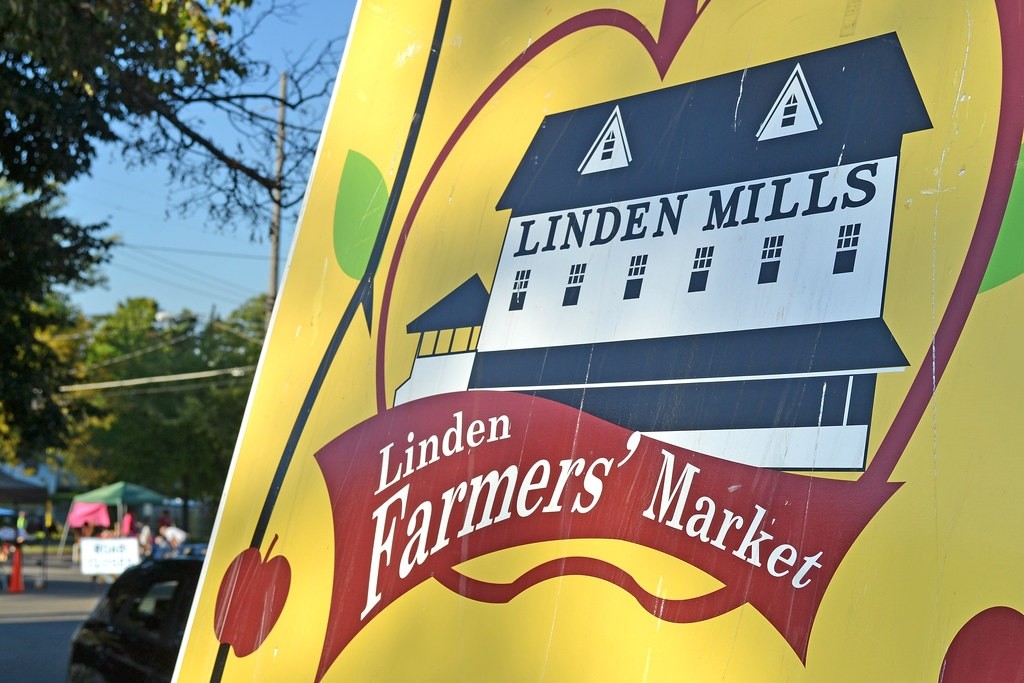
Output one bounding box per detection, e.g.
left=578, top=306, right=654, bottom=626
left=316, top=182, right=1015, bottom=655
left=17, top=512, right=32, bottom=540
left=44, top=500, right=57, bottom=540
left=122, top=507, right=193, bottom=556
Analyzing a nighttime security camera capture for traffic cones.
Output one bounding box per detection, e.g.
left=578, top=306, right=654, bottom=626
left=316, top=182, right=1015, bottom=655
left=6, top=549, right=26, bottom=593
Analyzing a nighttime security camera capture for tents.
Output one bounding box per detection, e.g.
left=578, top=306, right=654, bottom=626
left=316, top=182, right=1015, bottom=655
left=0, top=471, right=48, bottom=505
left=57, top=480, right=183, bottom=569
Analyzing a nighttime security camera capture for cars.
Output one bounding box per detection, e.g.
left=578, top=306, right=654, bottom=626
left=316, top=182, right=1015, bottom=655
left=64, top=552, right=204, bottom=683
left=142, top=542, right=209, bottom=567
